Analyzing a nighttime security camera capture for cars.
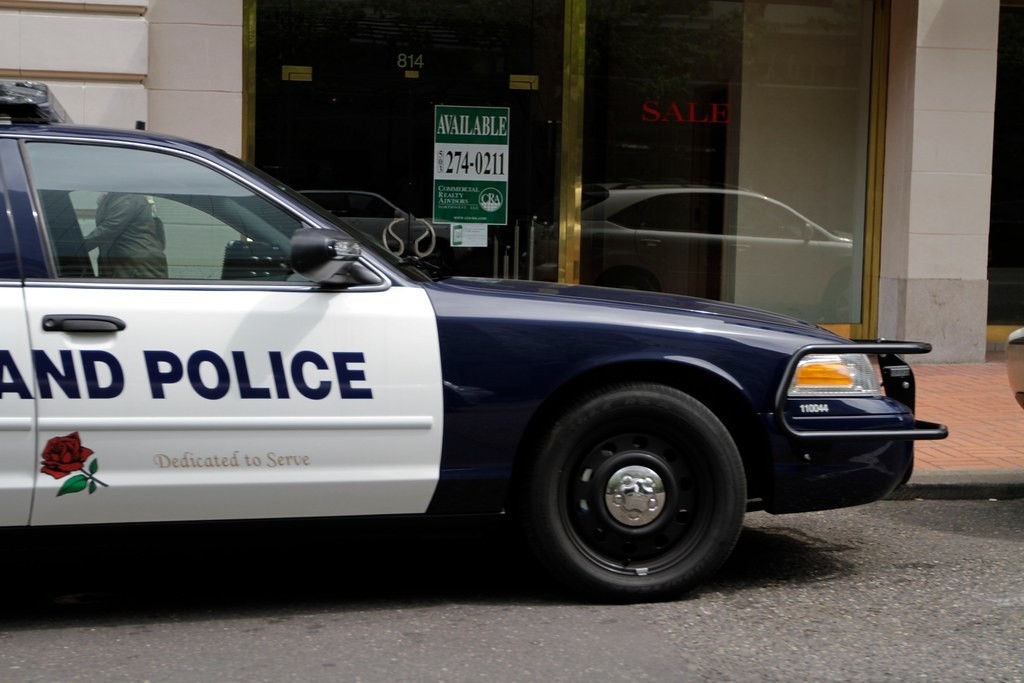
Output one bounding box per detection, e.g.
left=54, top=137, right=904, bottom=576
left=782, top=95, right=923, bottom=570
left=296, top=187, right=475, bottom=278
left=518, top=181, right=854, bottom=326
left=1, top=76, right=948, bottom=611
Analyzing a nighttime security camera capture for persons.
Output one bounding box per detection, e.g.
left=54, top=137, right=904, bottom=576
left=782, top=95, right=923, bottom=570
left=83, top=192, right=169, bottom=278
